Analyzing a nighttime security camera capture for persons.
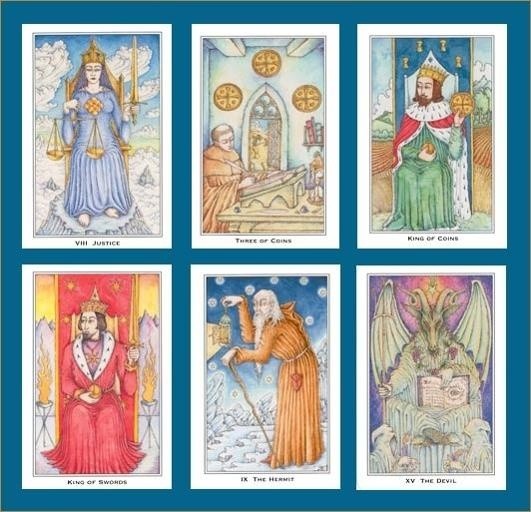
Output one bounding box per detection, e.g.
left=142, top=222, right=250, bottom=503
left=392, top=60, right=470, bottom=229
left=218, top=287, right=326, bottom=468
left=202, top=123, right=285, bottom=232
left=51, top=282, right=142, bottom=475
left=47, top=38, right=141, bottom=227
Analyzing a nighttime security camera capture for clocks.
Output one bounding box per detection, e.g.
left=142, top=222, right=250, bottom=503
left=451, top=91, right=474, bottom=118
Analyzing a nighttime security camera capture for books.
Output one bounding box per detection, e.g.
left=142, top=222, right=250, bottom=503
left=416, top=373, right=471, bottom=408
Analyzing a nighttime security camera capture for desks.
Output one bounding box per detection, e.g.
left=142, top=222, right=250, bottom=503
left=217, top=199, right=325, bottom=235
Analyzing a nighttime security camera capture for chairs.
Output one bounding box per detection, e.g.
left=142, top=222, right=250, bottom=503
left=403, top=50, right=459, bottom=114
left=70, top=309, right=121, bottom=346
left=64, top=62, right=131, bottom=191
